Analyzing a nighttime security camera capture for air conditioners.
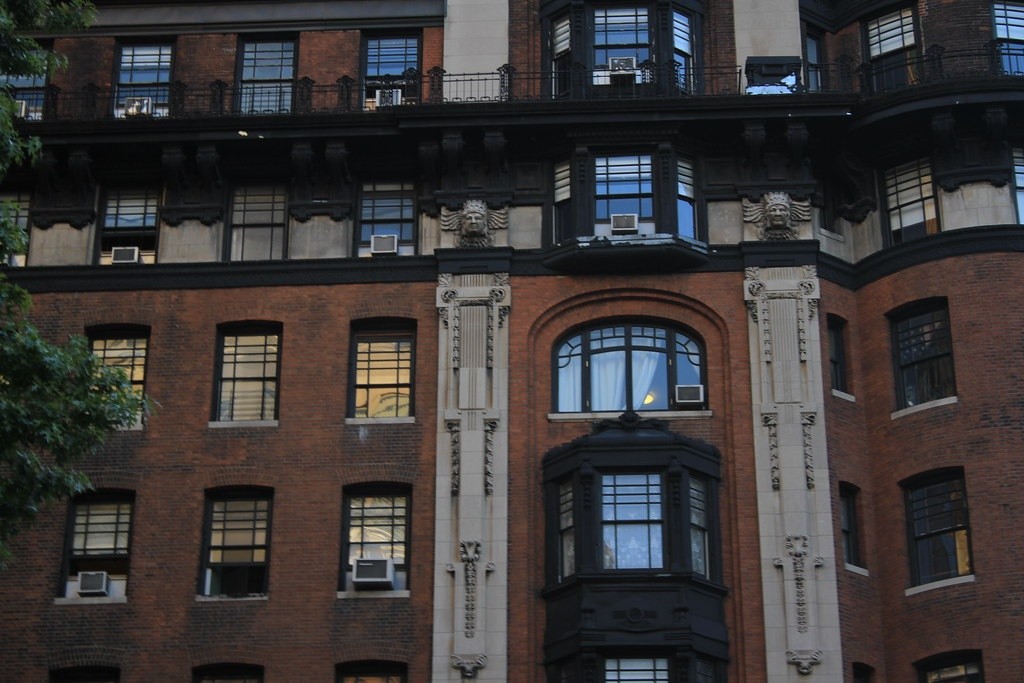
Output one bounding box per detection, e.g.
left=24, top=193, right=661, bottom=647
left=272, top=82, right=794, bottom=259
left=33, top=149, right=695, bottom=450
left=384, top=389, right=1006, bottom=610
left=675, top=385, right=705, bottom=410
left=352, top=558, right=395, bottom=591
left=609, top=57, right=636, bottom=83
left=370, top=234, right=398, bottom=256
left=13, top=100, right=27, bottom=119
left=78, top=571, right=110, bottom=597
left=125, top=97, right=152, bottom=118
left=375, top=89, right=402, bottom=110
left=610, top=214, right=638, bottom=235
left=111, top=247, right=140, bottom=263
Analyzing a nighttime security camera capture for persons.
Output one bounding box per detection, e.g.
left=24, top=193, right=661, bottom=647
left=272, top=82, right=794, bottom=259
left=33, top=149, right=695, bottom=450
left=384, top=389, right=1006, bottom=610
left=763, top=192, right=791, bottom=230
left=461, top=199, right=488, bottom=242
